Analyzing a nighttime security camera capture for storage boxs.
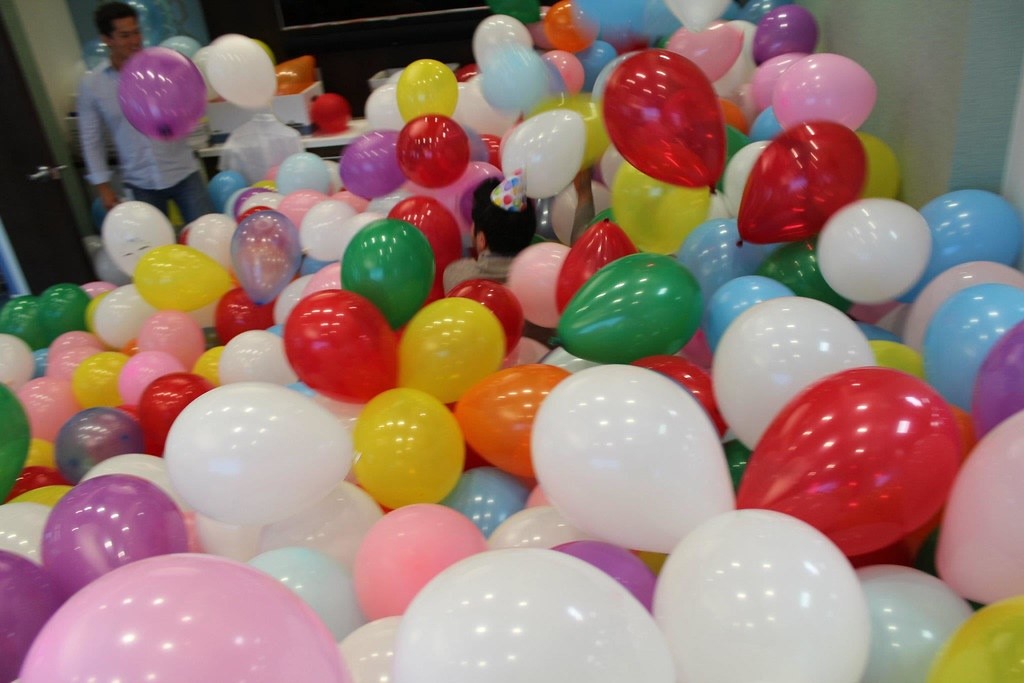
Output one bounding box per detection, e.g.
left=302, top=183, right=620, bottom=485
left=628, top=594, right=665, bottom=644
left=201, top=81, right=327, bottom=144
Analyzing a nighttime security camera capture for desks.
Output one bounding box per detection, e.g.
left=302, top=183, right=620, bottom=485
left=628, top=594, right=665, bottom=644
left=193, top=114, right=367, bottom=184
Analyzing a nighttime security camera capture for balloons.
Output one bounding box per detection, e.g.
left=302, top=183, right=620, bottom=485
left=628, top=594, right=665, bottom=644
left=0, top=0, right=1024, bottom=683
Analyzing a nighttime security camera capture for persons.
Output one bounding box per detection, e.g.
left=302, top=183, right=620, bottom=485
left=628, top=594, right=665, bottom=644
left=77, top=3, right=213, bottom=230
left=442, top=170, right=596, bottom=350
